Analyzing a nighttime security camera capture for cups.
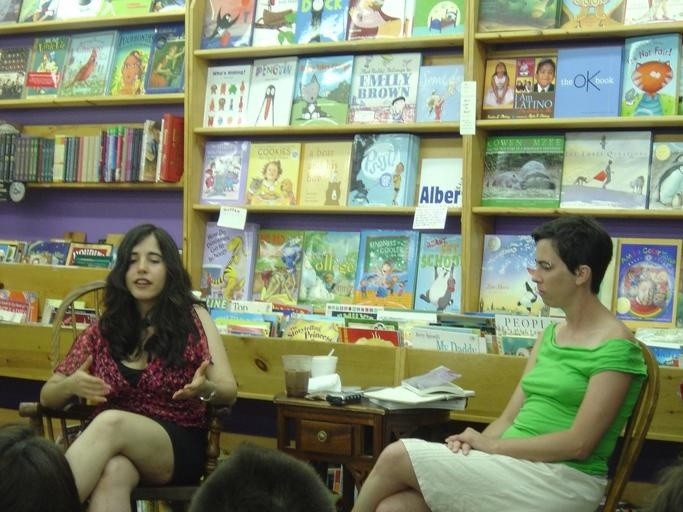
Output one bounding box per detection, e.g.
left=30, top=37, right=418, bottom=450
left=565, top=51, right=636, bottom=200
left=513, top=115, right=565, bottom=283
left=282, top=354, right=313, bottom=400
left=312, top=355, right=339, bottom=380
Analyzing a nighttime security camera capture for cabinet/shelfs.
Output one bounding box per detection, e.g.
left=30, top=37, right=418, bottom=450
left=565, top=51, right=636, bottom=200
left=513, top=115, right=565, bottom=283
left=0, top=0, right=187, bottom=335
left=471, top=2, right=677, bottom=371
left=187, top=2, right=469, bottom=340
left=276, top=393, right=451, bottom=512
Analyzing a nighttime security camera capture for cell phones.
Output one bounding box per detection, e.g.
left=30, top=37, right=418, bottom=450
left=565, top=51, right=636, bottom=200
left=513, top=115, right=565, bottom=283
left=326, top=393, right=361, bottom=405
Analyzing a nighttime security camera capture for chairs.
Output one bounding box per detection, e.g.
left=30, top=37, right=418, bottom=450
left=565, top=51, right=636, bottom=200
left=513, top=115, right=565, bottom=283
left=595, top=334, right=662, bottom=510
left=17, top=276, right=220, bottom=511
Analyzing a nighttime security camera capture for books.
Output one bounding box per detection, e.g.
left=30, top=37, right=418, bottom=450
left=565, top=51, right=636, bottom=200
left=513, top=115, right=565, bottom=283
left=480, top=228, right=683, bottom=328
left=477, top=33, right=682, bottom=119
left=1, top=231, right=125, bottom=331
left=199, top=1, right=463, bottom=48
left=634, top=327, right=683, bottom=367
left=360, top=363, right=476, bottom=412
left=481, top=130, right=683, bottom=214
left=474, top=0, right=682, bottom=35
left=0, top=28, right=183, bottom=97
left=201, top=134, right=462, bottom=209
left=206, top=300, right=560, bottom=353
left=1, top=1, right=186, bottom=24
left=198, top=219, right=461, bottom=314
left=201, top=51, right=465, bottom=124
left=0, top=114, right=184, bottom=184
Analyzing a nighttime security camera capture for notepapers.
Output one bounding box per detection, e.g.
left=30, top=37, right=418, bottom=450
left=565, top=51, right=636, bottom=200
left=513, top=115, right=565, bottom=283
left=216, top=206, right=248, bottom=232
left=412, top=202, right=448, bottom=229
left=459, top=81, right=476, bottom=137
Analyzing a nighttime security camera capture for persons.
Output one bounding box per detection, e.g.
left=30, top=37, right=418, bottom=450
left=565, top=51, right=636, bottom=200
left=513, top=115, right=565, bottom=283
left=190, top=442, right=339, bottom=512
left=1, top=417, right=87, bottom=511
left=354, top=215, right=648, bottom=511
left=41, top=223, right=237, bottom=512
